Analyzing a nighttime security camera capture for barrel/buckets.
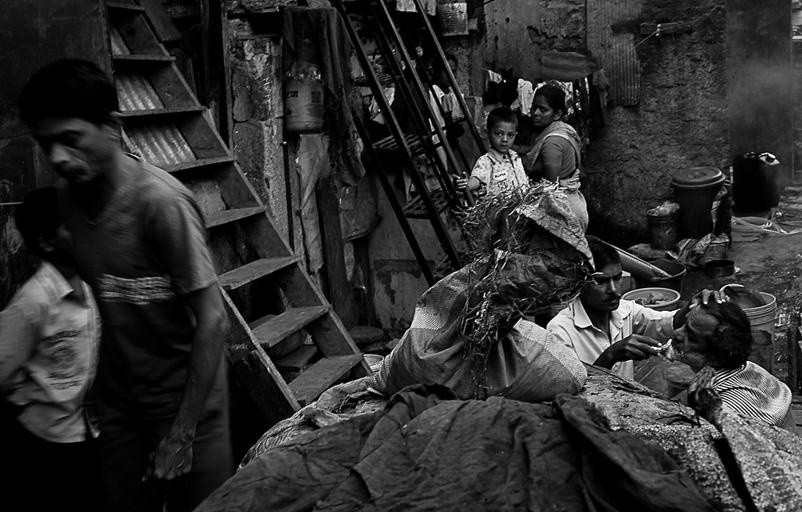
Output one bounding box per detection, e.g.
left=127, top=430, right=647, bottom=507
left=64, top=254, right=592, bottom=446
left=647, top=210, right=678, bottom=251
left=621, top=288, right=682, bottom=311
left=285, top=79, right=323, bottom=131
left=364, top=353, right=386, bottom=372
left=631, top=259, right=687, bottom=292
left=726, top=293, right=777, bottom=377
left=733, top=153, right=781, bottom=212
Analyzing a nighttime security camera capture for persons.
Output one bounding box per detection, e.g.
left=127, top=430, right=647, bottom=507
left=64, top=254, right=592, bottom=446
left=671, top=301, right=799, bottom=438
left=546, top=243, right=730, bottom=382
left=451, top=107, right=530, bottom=201
left=0, top=187, right=103, bottom=512
left=427, top=50, right=481, bottom=175
left=523, top=83, right=590, bottom=235
left=18, top=56, right=236, bottom=511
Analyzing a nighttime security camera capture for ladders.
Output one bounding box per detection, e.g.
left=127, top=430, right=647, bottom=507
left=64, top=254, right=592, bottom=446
left=315, top=0, right=490, bottom=292
left=103, top=0, right=372, bottom=424
